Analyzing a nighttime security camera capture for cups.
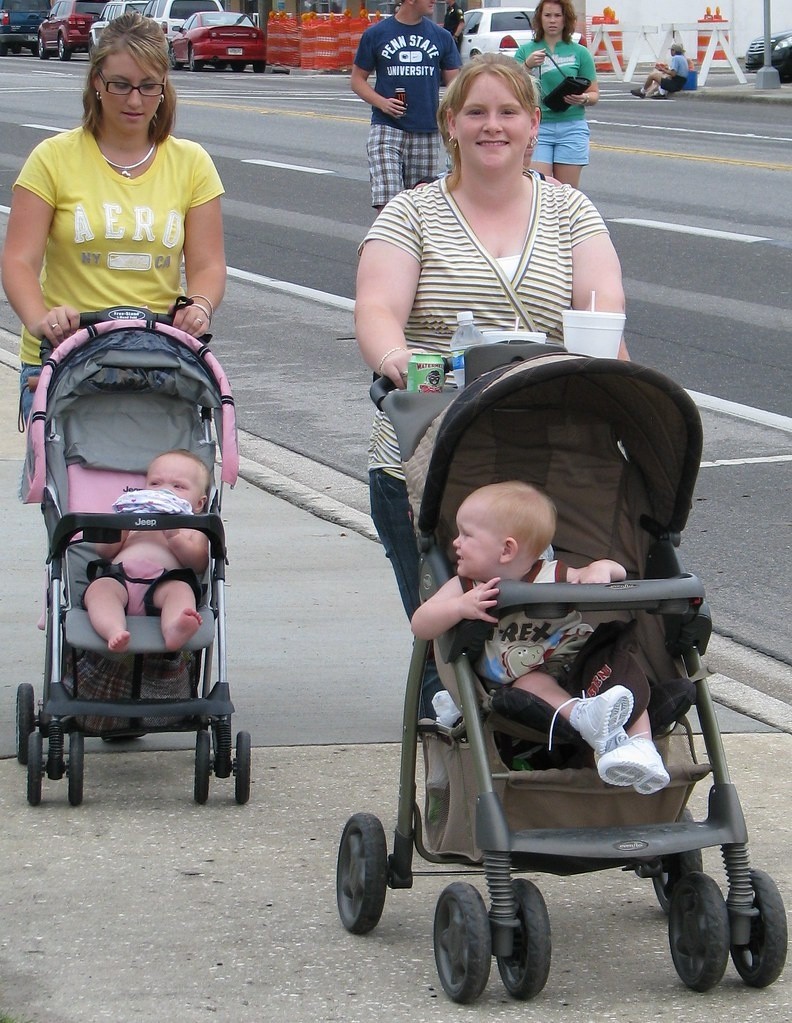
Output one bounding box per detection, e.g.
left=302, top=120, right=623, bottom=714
left=561, top=309, right=627, bottom=359
left=484, top=331, right=546, bottom=347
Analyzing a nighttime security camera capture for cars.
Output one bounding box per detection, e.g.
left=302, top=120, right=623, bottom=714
left=745, top=29, right=792, bottom=81
left=459, top=8, right=586, bottom=76
left=168, top=12, right=267, bottom=73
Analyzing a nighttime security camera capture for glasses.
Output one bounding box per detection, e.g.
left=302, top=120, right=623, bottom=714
left=98, top=68, right=167, bottom=96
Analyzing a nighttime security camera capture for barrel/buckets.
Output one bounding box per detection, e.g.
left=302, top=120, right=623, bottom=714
left=681, top=70, right=697, bottom=90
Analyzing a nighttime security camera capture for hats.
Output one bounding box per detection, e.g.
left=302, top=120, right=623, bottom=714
left=668, top=44, right=682, bottom=51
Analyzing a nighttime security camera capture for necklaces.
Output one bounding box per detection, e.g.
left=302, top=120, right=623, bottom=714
left=101, top=140, right=157, bottom=178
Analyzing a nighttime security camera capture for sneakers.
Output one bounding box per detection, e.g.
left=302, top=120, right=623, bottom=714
left=597, top=732, right=669, bottom=795
left=631, top=88, right=645, bottom=98
left=550, top=685, right=634, bottom=749
left=652, top=91, right=666, bottom=99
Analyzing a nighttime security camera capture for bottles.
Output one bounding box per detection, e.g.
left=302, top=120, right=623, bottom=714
left=395, top=88, right=406, bottom=107
left=449, top=311, right=489, bottom=387
left=511, top=736, right=556, bottom=771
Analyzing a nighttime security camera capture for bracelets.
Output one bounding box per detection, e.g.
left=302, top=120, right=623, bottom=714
left=187, top=295, right=213, bottom=319
left=379, top=347, right=408, bottom=376
left=580, top=93, right=589, bottom=105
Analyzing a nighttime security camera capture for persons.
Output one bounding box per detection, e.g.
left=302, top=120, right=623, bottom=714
left=352, top=52, right=632, bottom=730
left=413, top=74, right=564, bottom=191
left=0, top=11, right=227, bottom=631
left=631, top=43, right=695, bottom=99
left=351, top=0, right=464, bottom=216
left=82, top=449, right=211, bottom=662
left=410, top=479, right=672, bottom=796
left=511, top=0, right=600, bottom=189
left=443, top=0, right=465, bottom=56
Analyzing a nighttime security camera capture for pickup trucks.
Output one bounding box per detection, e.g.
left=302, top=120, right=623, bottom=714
left=0, top=0, right=52, bottom=56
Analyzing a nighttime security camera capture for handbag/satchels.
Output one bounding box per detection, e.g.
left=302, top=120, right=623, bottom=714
left=539, top=54, right=591, bottom=112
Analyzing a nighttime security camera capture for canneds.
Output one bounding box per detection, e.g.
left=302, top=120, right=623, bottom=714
left=406, top=352, right=444, bottom=394
left=395, top=87, right=407, bottom=116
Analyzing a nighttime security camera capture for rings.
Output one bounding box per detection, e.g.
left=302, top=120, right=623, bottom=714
left=196, top=319, right=203, bottom=325
left=51, top=323, right=58, bottom=330
left=401, top=370, right=408, bottom=377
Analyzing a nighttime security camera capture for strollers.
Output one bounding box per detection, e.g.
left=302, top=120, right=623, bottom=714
left=336, top=340, right=787, bottom=1005
left=14, top=305, right=252, bottom=805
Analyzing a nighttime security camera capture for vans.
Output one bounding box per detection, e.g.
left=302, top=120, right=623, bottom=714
left=141, top=0, right=227, bottom=46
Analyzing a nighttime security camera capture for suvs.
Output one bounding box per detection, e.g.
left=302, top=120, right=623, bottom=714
left=88, top=0, right=150, bottom=61
left=38, top=0, right=114, bottom=61
